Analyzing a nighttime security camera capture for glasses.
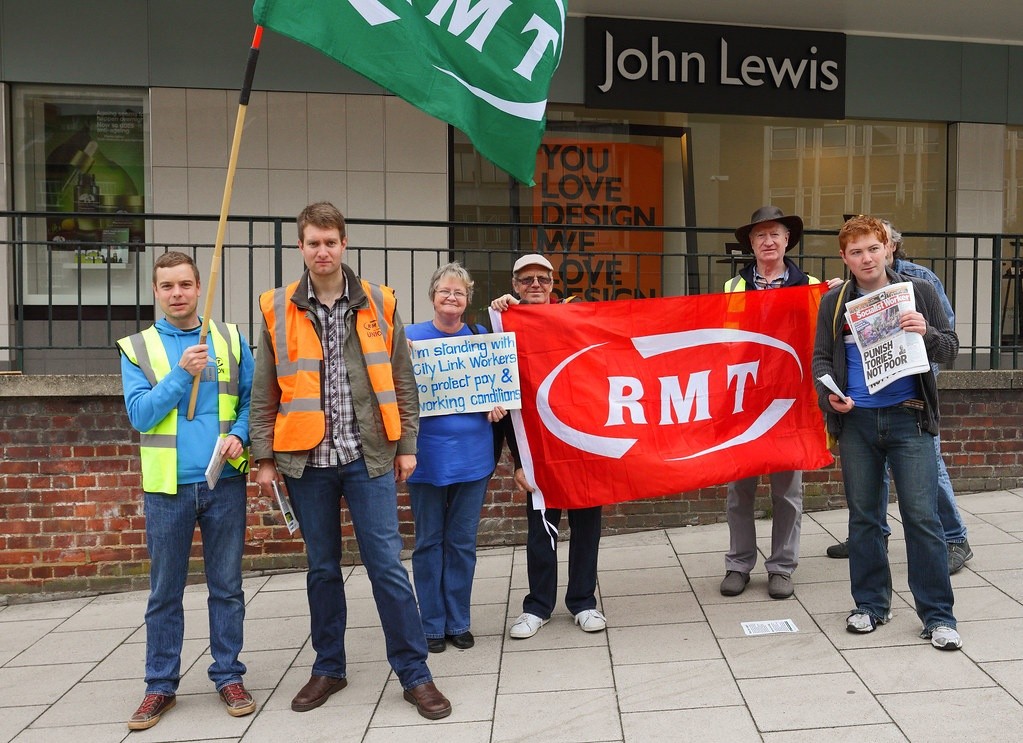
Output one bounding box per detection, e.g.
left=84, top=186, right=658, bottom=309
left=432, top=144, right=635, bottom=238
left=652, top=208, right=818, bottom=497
left=754, top=266, right=786, bottom=288
left=518, top=277, right=551, bottom=285
left=434, top=289, right=468, bottom=297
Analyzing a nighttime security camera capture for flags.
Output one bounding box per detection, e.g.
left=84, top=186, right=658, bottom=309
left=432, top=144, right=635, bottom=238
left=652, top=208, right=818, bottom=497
left=253, top=0, right=570, bottom=188
left=490, top=281, right=835, bottom=510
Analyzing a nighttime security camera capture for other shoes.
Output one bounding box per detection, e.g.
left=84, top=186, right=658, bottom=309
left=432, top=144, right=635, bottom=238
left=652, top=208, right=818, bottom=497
left=827, top=536, right=889, bottom=558
left=720, top=571, right=750, bottom=596
left=768, top=573, right=794, bottom=598
left=948, top=541, right=973, bottom=575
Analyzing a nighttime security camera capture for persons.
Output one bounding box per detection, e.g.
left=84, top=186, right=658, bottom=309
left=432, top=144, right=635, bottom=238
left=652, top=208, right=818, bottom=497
left=810, top=215, right=962, bottom=652
left=488, top=253, right=608, bottom=639
left=719, top=206, right=844, bottom=598
left=403, top=262, right=509, bottom=654
left=249, top=195, right=454, bottom=721
left=825, top=216, right=974, bottom=576
left=116, top=251, right=256, bottom=731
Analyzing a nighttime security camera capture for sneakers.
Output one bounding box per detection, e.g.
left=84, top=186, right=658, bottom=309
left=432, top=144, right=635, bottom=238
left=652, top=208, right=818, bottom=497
left=572, top=609, right=607, bottom=632
left=509, top=613, right=551, bottom=638
left=219, top=683, right=256, bottom=716
left=846, top=613, right=893, bottom=634
left=128, top=694, right=176, bottom=729
left=922, top=623, right=963, bottom=650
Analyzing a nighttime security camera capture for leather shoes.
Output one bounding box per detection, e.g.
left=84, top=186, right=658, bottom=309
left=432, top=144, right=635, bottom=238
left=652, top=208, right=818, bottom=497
left=403, top=681, right=452, bottom=720
left=290, top=675, right=347, bottom=712
left=427, top=638, right=446, bottom=653
left=450, top=631, right=475, bottom=649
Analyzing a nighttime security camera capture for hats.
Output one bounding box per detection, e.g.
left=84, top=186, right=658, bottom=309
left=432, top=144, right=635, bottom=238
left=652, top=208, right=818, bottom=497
left=513, top=254, right=554, bottom=275
left=735, top=206, right=803, bottom=254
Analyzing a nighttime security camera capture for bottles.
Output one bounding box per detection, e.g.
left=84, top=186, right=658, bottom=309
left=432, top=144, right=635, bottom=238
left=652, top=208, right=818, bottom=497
left=74, top=173, right=101, bottom=236
left=122, top=195, right=145, bottom=235
left=99, top=194, right=123, bottom=230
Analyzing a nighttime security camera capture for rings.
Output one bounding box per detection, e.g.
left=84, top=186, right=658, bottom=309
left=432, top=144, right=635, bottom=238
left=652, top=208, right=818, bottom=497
left=234, top=452, right=237, bottom=454
left=401, top=480, right=406, bottom=483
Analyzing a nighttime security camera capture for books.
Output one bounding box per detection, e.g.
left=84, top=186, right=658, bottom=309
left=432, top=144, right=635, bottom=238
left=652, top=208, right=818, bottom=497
left=204, top=436, right=227, bottom=490
